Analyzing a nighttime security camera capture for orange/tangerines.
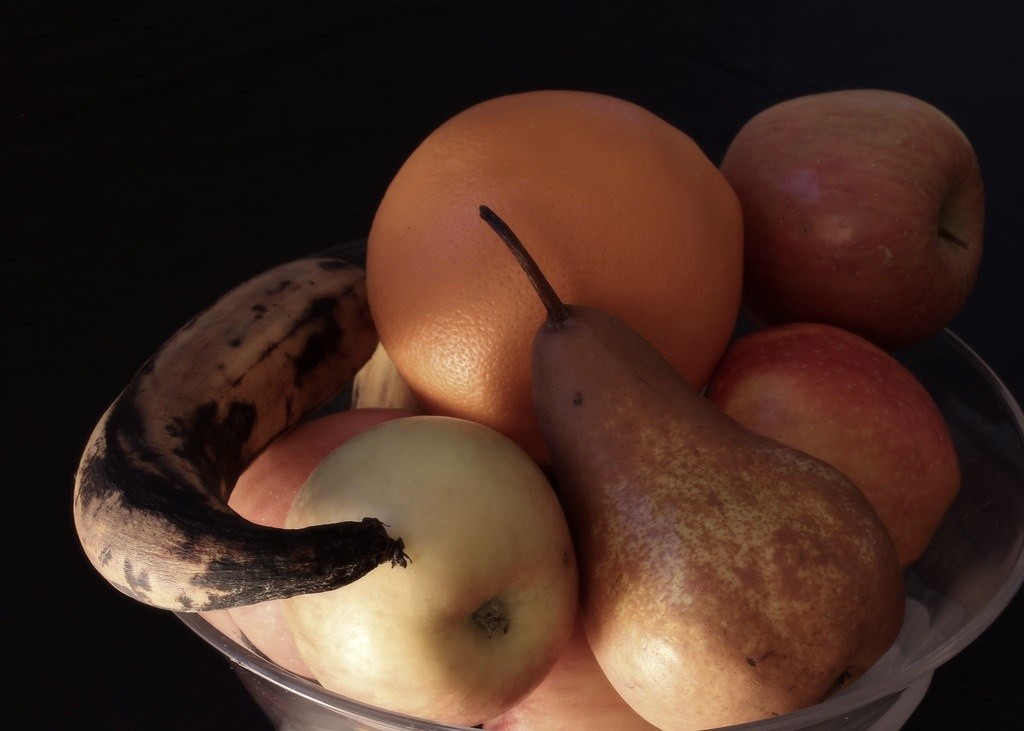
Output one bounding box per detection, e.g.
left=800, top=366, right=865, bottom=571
left=369, top=93, right=748, bottom=460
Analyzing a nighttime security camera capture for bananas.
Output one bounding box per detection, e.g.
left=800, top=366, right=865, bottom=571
left=73, top=257, right=405, bottom=608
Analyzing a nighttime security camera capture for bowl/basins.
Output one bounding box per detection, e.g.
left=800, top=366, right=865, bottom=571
left=169, top=326, right=1024, bottom=731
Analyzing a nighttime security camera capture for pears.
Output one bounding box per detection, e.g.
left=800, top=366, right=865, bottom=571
left=473, top=205, right=909, bottom=731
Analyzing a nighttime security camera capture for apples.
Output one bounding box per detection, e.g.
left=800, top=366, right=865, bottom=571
left=221, top=407, right=411, bottom=677
left=482, top=636, right=657, bottom=731
left=284, top=418, right=577, bottom=727
left=723, top=91, right=985, bottom=339
left=712, top=326, right=960, bottom=562
